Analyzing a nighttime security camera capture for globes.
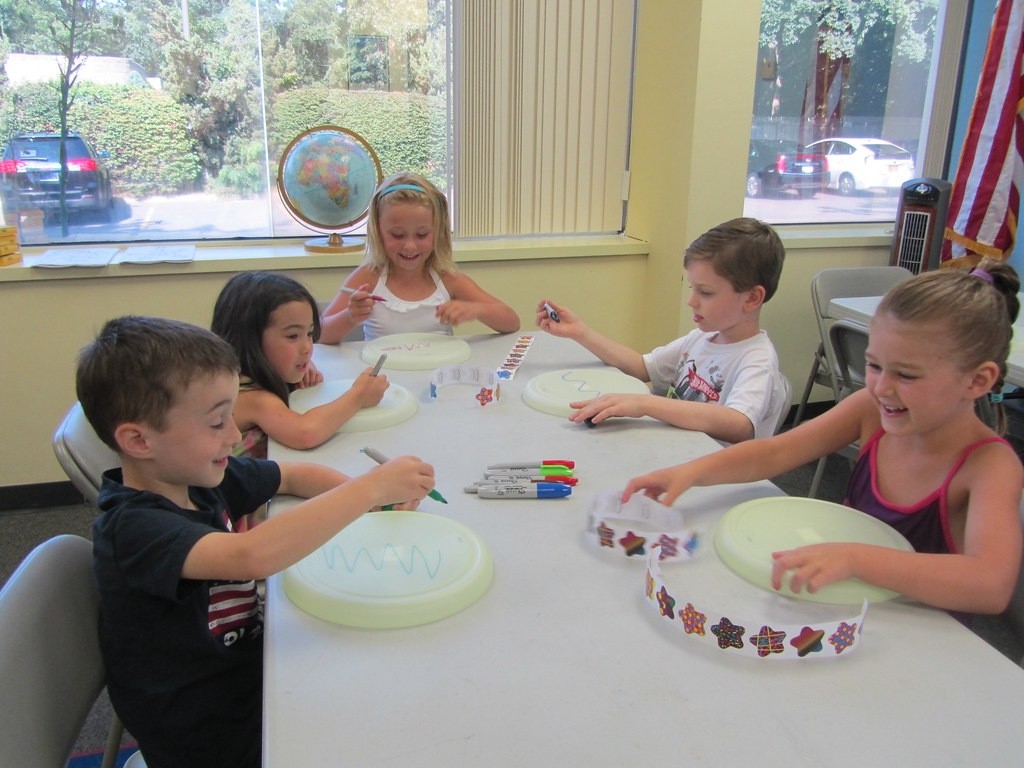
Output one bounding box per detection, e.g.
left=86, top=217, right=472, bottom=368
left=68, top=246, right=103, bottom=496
left=275, top=125, right=385, bottom=254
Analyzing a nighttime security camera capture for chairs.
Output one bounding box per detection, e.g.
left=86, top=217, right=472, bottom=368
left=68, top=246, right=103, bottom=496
left=53, top=400, right=123, bottom=504
left=791, top=267, right=993, bottom=436
left=1, top=535, right=123, bottom=768
left=808, top=317, right=871, bottom=500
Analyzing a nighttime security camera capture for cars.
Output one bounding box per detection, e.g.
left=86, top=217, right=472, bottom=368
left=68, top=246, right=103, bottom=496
left=747, top=140, right=830, bottom=200
left=805, top=137, right=915, bottom=196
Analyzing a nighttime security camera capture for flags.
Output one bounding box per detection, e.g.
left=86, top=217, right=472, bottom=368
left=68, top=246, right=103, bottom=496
left=795, top=0, right=850, bottom=220
left=940, top=0, right=1024, bottom=274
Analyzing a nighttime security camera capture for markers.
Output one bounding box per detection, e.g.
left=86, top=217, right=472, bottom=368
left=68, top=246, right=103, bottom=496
left=370, top=353, right=388, bottom=377
left=463, top=459, right=578, bottom=499
left=364, top=445, right=449, bottom=506
left=340, top=287, right=388, bottom=302
left=544, top=304, right=560, bottom=324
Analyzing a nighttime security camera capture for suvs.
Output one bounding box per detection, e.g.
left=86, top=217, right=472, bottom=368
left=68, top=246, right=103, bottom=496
left=0, top=133, right=113, bottom=225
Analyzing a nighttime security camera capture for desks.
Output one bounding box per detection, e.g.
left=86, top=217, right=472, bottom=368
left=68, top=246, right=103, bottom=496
left=828, top=292, right=1024, bottom=389
left=261, top=332, right=1023, bottom=768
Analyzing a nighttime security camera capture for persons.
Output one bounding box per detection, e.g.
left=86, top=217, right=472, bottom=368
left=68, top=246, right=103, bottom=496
left=622, top=263, right=1023, bottom=614
left=211, top=270, right=391, bottom=529
left=536, top=218, right=784, bottom=445
left=76, top=315, right=434, bottom=768
left=316, top=172, right=520, bottom=344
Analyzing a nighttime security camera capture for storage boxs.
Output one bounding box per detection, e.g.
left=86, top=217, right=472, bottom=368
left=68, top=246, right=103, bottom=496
left=0, top=226, right=22, bottom=267
